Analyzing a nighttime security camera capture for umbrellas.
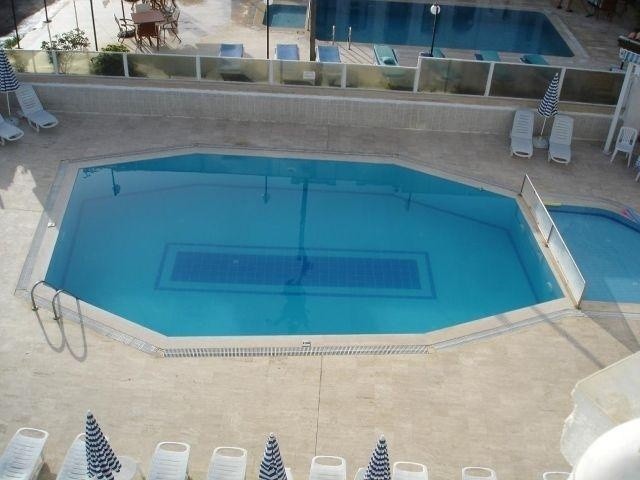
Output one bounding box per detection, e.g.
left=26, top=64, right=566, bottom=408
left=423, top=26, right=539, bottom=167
left=536, top=72, right=560, bottom=136
left=364, top=435, right=391, bottom=480
left=0, top=47, right=20, bottom=119
left=85, top=409, right=122, bottom=480
left=258, top=433, right=287, bottom=480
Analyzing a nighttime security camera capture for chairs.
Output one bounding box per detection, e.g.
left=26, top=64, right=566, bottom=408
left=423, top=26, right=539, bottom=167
left=218, top=43, right=550, bottom=82
left=548, top=115, right=574, bottom=166
left=56, top=433, right=108, bottom=479
left=543, top=472, right=571, bottom=478
left=1, top=428, right=49, bottom=479
left=114, top=0, right=182, bottom=51
left=0, top=113, right=24, bottom=146
left=392, top=462, right=430, bottom=479
left=510, top=109, right=535, bottom=160
left=146, top=443, right=191, bottom=479
left=206, top=444, right=247, bottom=477
left=15, top=83, right=59, bottom=133
left=462, top=467, right=496, bottom=477
left=610, top=126, right=638, bottom=168
left=309, top=457, right=346, bottom=479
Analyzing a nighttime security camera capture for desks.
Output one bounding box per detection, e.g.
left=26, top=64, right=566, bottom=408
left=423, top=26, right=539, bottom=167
left=113, top=456, right=144, bottom=480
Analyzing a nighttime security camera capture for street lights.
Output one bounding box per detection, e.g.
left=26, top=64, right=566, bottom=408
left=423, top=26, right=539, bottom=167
left=265, top=0, right=276, bottom=60
left=430, top=1, right=442, bottom=56
